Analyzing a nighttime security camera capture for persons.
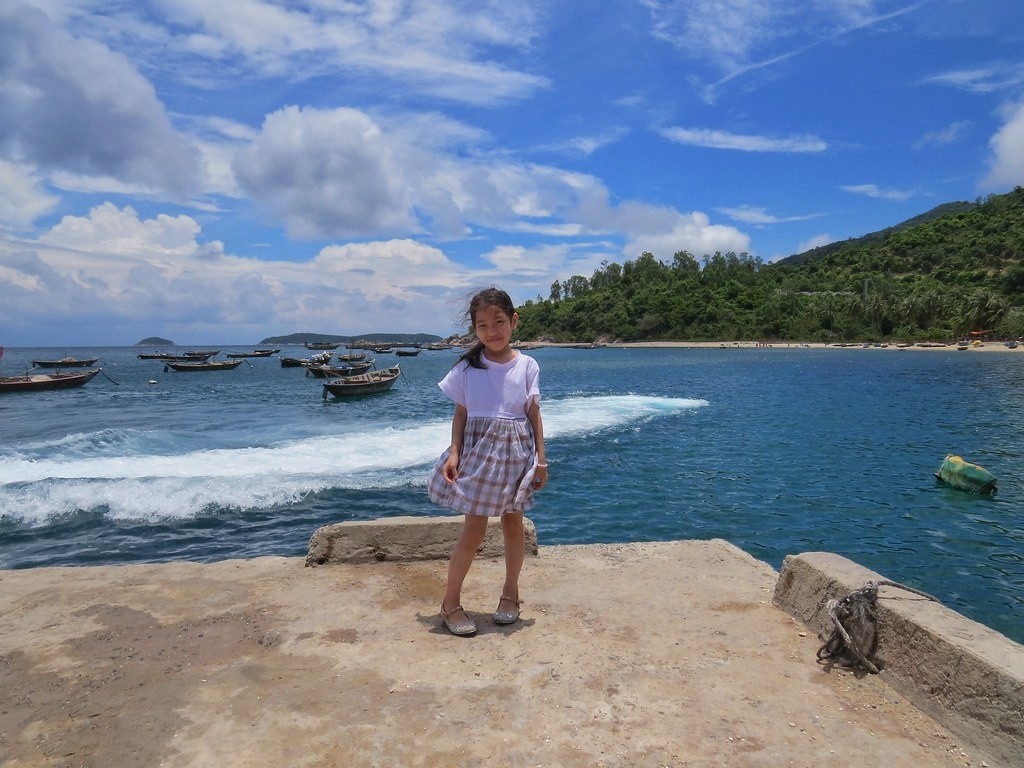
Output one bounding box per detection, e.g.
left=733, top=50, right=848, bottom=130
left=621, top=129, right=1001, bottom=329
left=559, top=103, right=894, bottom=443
left=426, top=288, right=550, bottom=636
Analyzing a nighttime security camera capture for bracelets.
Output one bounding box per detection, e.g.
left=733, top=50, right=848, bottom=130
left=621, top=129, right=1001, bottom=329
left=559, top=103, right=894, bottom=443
left=537, top=463, right=548, bottom=468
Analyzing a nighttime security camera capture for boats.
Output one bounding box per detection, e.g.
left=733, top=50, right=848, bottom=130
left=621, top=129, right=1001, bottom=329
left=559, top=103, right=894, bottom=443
left=305, top=342, right=340, bottom=350
left=137, top=352, right=213, bottom=361
left=322, top=363, right=401, bottom=401
left=0, top=368, right=103, bottom=392
left=184, top=349, right=222, bottom=356
left=227, top=351, right=274, bottom=358
left=254, top=349, right=282, bottom=354
left=166, top=360, right=247, bottom=371
left=281, top=351, right=337, bottom=368
left=307, top=358, right=376, bottom=379
left=337, top=353, right=367, bottom=361
left=31, top=354, right=102, bottom=367
left=395, top=348, right=424, bottom=357
left=370, top=346, right=394, bottom=354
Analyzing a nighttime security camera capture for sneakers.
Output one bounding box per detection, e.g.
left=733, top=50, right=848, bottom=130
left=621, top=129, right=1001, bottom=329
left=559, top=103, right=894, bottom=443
left=439, top=602, right=477, bottom=636
left=492, top=592, right=525, bottom=625
